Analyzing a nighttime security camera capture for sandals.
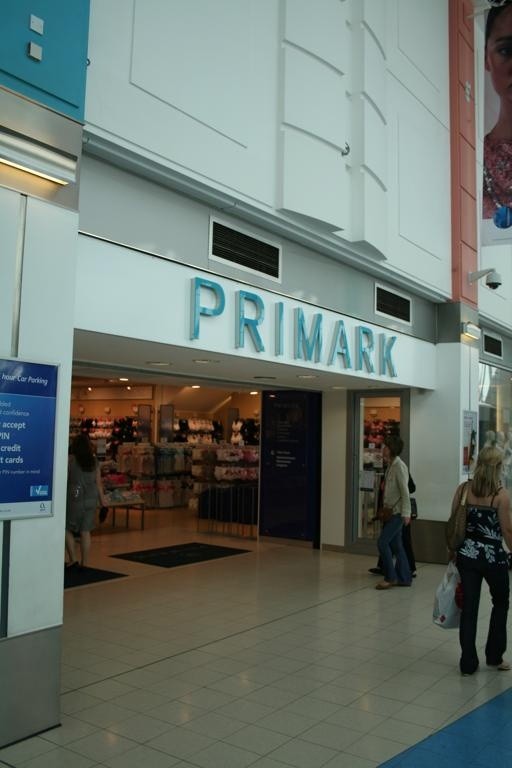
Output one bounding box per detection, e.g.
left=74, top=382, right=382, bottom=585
left=463, top=671, right=472, bottom=676
left=487, top=661, right=511, bottom=670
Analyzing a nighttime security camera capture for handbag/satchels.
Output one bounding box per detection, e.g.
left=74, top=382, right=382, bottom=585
left=66, top=475, right=82, bottom=512
left=446, top=482, right=470, bottom=553
left=376, top=509, right=393, bottom=522
left=410, top=498, right=418, bottom=519
left=433, top=561, right=461, bottom=629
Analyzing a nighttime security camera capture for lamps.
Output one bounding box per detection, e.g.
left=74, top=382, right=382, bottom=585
left=462, top=320, right=482, bottom=340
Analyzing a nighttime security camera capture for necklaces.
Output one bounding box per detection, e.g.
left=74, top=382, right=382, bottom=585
left=484, top=166, right=512, bottom=228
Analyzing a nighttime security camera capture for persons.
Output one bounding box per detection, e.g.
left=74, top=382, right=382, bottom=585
left=370, top=436, right=416, bottom=589
left=483, top=0, right=512, bottom=219
left=484, top=431, right=512, bottom=489
left=65, top=435, right=107, bottom=568
left=446, top=442, right=512, bottom=676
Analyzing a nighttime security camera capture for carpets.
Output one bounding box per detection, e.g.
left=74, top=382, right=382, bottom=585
left=64, top=558, right=128, bottom=592
left=107, top=539, right=254, bottom=570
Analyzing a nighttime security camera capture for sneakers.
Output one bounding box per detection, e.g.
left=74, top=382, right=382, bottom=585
left=368, top=567, right=416, bottom=590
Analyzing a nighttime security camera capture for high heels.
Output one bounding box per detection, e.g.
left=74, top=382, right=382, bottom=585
left=66, top=561, right=88, bottom=574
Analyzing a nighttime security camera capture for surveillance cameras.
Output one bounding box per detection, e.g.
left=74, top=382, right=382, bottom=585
left=484, top=271, right=502, bottom=290
